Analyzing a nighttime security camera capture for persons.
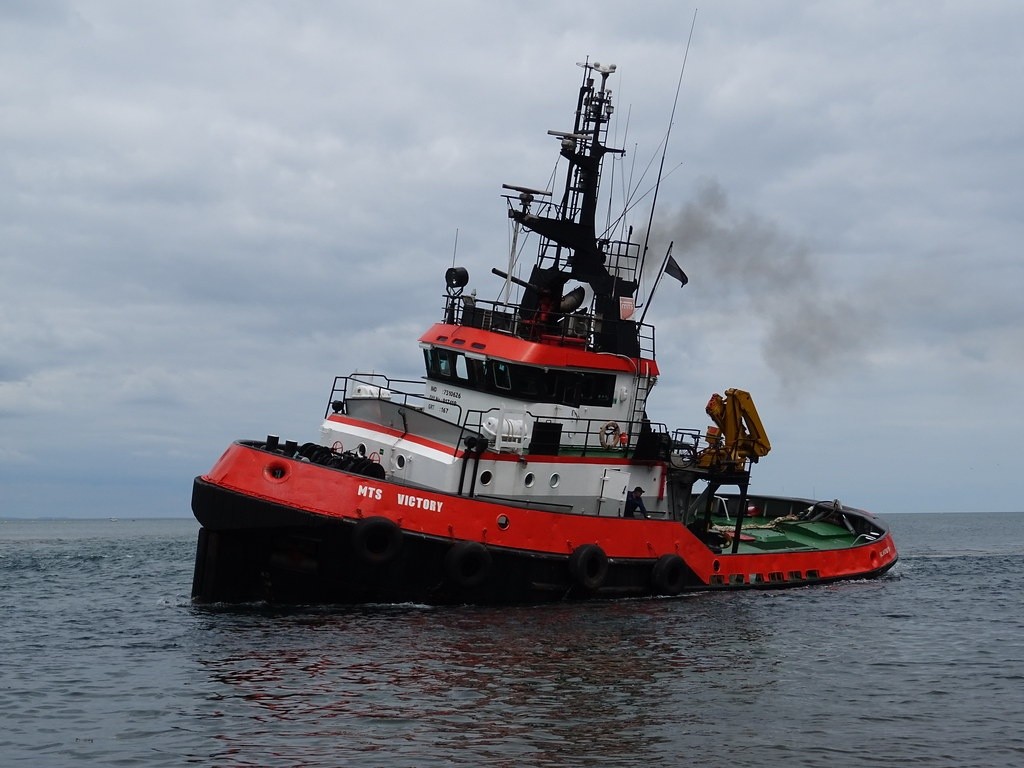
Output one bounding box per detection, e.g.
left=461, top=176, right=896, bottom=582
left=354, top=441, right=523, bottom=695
left=620, top=486, right=652, bottom=522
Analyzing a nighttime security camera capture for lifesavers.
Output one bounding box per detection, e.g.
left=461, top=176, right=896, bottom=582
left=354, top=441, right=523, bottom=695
left=599, top=421, right=620, bottom=447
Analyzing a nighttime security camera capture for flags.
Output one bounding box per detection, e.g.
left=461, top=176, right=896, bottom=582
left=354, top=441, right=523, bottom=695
left=664, top=254, right=688, bottom=288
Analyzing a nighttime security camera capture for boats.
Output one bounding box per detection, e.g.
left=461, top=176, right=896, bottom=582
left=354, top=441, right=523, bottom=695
left=192, top=6, right=900, bottom=603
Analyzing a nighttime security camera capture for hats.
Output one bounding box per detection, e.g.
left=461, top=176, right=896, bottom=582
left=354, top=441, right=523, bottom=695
left=635, top=487, right=645, bottom=493
